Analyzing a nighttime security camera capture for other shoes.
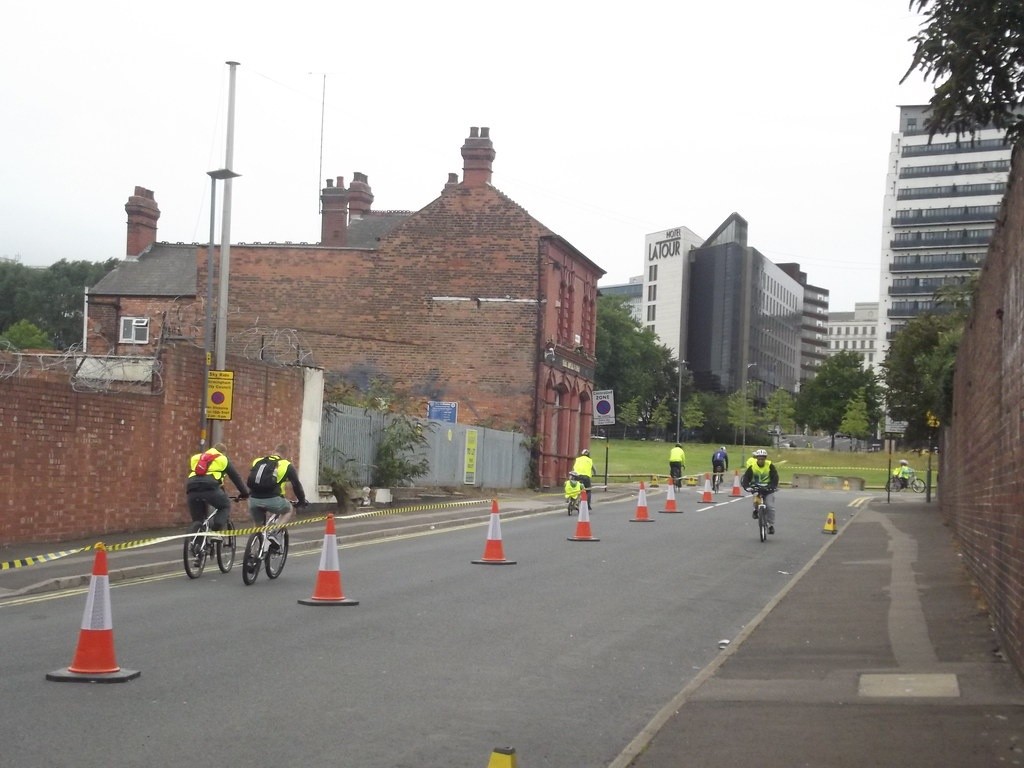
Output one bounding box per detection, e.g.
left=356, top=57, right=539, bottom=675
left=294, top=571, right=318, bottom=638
left=588, top=504, right=592, bottom=510
left=768, top=526, right=775, bottom=535
left=247, top=560, right=256, bottom=573
left=192, top=557, right=204, bottom=568
left=268, top=531, right=281, bottom=548
left=678, top=482, right=682, bottom=487
left=211, top=534, right=225, bottom=541
left=712, top=488, right=715, bottom=491
left=752, top=508, right=758, bottom=520
left=719, top=477, right=723, bottom=483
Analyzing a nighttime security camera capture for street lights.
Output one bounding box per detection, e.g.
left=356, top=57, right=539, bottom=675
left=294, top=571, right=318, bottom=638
left=677, top=359, right=690, bottom=442
left=740, top=363, right=757, bottom=466
left=197, top=167, right=244, bottom=451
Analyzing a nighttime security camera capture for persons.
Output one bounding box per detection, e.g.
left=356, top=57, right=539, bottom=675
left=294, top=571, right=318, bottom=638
left=892, top=461, right=915, bottom=489
left=187, top=442, right=249, bottom=566
left=573, top=450, right=597, bottom=510
left=564, top=471, right=585, bottom=502
left=669, top=443, right=685, bottom=488
left=743, top=449, right=780, bottom=533
left=712, top=448, right=728, bottom=490
left=249, top=444, right=309, bottom=548
left=806, top=441, right=811, bottom=448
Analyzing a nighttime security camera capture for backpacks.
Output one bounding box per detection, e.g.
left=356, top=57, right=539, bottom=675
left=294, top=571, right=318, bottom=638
left=246, top=456, right=278, bottom=492
left=195, top=452, right=220, bottom=477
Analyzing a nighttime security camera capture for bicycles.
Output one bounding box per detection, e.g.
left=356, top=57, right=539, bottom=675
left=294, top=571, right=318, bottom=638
left=241, top=500, right=309, bottom=586
left=183, top=496, right=247, bottom=579
left=712, top=468, right=727, bottom=494
left=885, top=469, right=927, bottom=493
left=745, top=484, right=778, bottom=542
left=672, top=465, right=686, bottom=494
left=568, top=475, right=594, bottom=516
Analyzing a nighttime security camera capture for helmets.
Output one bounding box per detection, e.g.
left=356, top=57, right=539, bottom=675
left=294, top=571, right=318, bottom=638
left=900, top=460, right=908, bottom=464
left=581, top=448, right=590, bottom=455
left=569, top=471, right=578, bottom=477
left=720, top=446, right=727, bottom=451
left=675, top=443, right=683, bottom=447
left=752, top=449, right=768, bottom=456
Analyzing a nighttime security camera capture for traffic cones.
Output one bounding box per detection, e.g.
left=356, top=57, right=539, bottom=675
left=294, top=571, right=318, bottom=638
left=487, top=744, right=517, bottom=768
left=727, top=470, right=746, bottom=497
left=296, top=513, right=360, bottom=607
left=697, top=472, right=718, bottom=503
left=628, top=481, right=655, bottom=522
left=471, top=497, right=518, bottom=565
left=841, top=479, right=851, bottom=491
left=566, top=489, right=601, bottom=541
left=821, top=510, right=839, bottom=535
left=46, top=549, right=141, bottom=684
left=657, top=476, right=684, bottom=513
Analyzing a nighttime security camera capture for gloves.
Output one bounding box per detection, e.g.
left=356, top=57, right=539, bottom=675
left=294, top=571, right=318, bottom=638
left=241, top=492, right=248, bottom=498
left=298, top=500, right=308, bottom=506
left=769, top=487, right=776, bottom=492
left=746, top=488, right=753, bottom=492
